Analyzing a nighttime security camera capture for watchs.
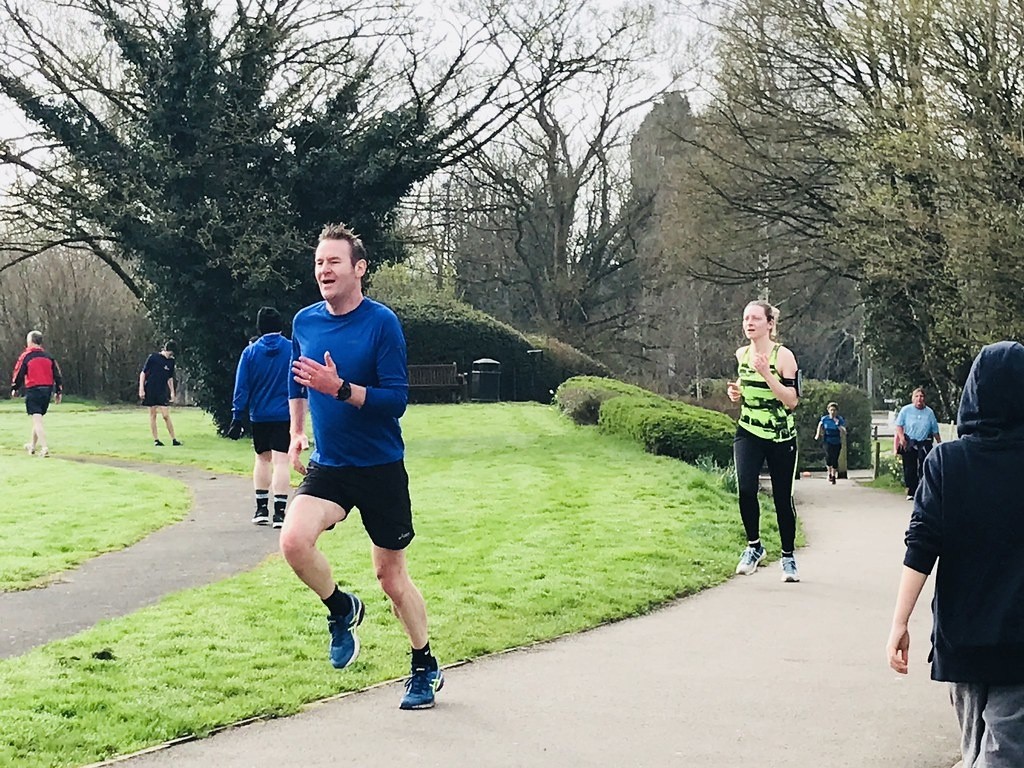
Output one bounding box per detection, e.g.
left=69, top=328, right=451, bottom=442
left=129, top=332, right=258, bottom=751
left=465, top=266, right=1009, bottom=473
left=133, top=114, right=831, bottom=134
left=337, top=380, right=351, bottom=400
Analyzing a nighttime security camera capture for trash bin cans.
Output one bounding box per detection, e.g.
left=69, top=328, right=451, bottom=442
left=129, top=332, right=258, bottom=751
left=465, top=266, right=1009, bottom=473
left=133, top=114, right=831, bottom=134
left=471, top=359, right=502, bottom=403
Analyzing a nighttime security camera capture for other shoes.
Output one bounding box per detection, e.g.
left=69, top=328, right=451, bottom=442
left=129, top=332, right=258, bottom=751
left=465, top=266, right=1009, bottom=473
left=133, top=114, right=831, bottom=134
left=906, top=495, right=914, bottom=500
left=41, top=448, right=50, bottom=456
left=832, top=476, right=836, bottom=484
left=828, top=474, right=832, bottom=481
left=155, top=441, right=164, bottom=446
left=174, top=441, right=182, bottom=445
left=24, top=443, right=35, bottom=454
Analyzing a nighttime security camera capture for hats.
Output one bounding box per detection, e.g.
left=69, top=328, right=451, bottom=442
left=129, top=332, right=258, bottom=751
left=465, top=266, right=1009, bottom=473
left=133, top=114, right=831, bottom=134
left=256, top=307, right=282, bottom=335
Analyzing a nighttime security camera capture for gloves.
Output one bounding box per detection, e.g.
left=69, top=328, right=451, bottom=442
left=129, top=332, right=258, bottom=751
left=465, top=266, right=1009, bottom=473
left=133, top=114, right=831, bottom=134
left=228, top=418, right=243, bottom=440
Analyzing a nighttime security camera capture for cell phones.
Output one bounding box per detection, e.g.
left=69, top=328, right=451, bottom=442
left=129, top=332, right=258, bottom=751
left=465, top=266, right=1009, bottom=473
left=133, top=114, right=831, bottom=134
left=797, top=370, right=803, bottom=396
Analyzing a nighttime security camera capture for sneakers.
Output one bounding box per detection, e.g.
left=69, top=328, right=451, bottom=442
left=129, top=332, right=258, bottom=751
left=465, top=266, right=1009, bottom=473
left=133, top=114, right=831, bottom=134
left=327, top=594, right=365, bottom=669
left=272, top=514, right=284, bottom=527
left=735, top=546, right=767, bottom=575
left=398, top=656, right=445, bottom=710
left=251, top=507, right=269, bottom=525
left=781, top=557, right=800, bottom=583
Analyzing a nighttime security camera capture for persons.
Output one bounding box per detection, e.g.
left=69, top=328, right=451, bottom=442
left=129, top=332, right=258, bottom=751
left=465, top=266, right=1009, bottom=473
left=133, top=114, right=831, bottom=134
left=885, top=341, right=1024, bottom=768
left=11, top=330, right=63, bottom=457
left=139, top=342, right=182, bottom=446
left=894, top=388, right=941, bottom=500
left=726, top=300, right=800, bottom=582
left=815, top=402, right=846, bottom=484
left=228, top=307, right=292, bottom=528
left=276, top=222, right=444, bottom=711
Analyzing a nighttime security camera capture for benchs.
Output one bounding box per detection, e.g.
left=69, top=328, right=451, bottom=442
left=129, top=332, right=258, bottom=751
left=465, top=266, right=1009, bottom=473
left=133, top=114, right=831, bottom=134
left=407, top=363, right=466, bottom=402
